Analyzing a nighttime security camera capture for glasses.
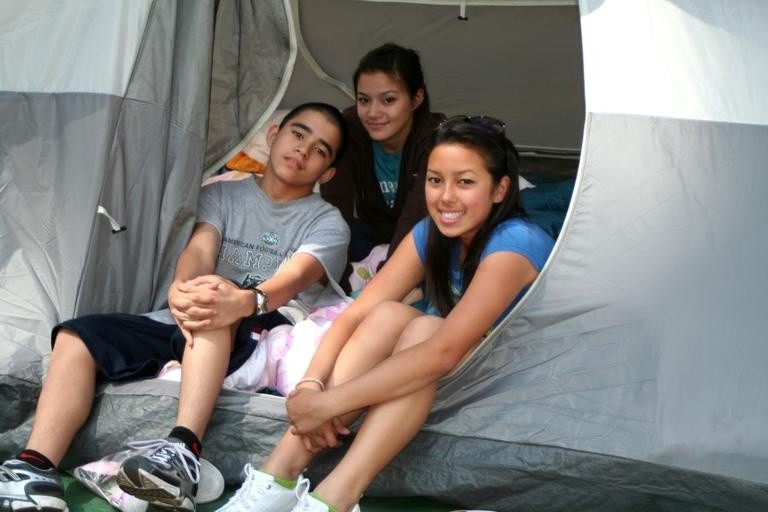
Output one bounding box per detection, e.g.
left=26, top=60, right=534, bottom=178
left=440, top=115, right=505, bottom=137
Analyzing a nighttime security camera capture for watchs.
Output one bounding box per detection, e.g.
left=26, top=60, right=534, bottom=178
left=250, top=285, right=269, bottom=321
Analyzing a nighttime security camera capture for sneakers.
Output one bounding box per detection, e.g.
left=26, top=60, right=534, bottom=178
left=0, top=458, right=69, bottom=512
left=214, top=464, right=361, bottom=511
left=117, top=437, right=200, bottom=512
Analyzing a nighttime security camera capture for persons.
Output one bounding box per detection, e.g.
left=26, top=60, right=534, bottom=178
left=0, top=103, right=352, bottom=512
left=213, top=116, right=554, bottom=511
left=318, top=42, right=445, bottom=272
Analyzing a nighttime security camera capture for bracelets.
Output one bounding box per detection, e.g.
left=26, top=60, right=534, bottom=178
left=294, top=377, right=324, bottom=390
